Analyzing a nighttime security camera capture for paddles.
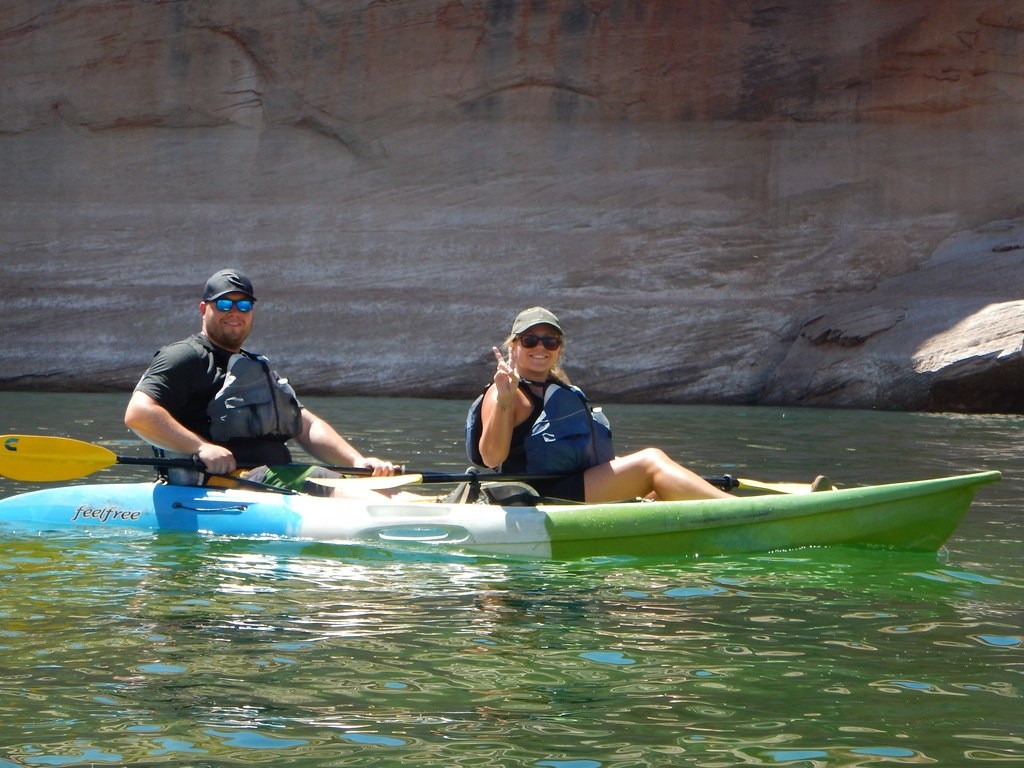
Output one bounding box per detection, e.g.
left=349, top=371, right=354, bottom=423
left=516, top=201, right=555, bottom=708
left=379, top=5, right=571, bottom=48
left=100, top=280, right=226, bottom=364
left=303, top=473, right=839, bottom=493
left=1, top=434, right=453, bottom=482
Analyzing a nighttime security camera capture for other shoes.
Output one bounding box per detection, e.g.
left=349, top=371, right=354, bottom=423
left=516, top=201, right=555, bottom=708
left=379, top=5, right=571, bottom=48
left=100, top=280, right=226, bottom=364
left=811, top=474, right=833, bottom=491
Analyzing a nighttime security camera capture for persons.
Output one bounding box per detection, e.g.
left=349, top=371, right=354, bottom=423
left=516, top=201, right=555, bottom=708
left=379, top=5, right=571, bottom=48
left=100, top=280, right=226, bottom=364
left=465, top=307, right=832, bottom=505
left=123, top=269, right=480, bottom=504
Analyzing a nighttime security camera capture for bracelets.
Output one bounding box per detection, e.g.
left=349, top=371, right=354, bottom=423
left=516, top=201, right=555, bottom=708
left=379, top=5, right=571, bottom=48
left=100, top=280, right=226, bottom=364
left=496, top=399, right=512, bottom=408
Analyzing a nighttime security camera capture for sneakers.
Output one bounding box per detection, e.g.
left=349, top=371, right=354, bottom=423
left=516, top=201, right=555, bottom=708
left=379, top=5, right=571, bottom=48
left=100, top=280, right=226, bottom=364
left=434, top=466, right=481, bottom=502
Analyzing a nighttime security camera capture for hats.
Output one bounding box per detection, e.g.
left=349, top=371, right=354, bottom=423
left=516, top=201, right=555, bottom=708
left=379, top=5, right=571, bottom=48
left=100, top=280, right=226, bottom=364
left=511, top=307, right=564, bottom=334
left=203, top=268, right=256, bottom=301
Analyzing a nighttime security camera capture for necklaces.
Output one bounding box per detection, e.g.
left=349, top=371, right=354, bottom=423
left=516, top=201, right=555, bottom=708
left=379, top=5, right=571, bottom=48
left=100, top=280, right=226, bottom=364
left=525, top=378, right=546, bottom=386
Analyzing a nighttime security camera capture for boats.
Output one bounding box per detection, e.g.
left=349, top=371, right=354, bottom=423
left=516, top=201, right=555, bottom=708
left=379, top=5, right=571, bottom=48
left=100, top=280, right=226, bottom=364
left=0, top=470, right=1004, bottom=557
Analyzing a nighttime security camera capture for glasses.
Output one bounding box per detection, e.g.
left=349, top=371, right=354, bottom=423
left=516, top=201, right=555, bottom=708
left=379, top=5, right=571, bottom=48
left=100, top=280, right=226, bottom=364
left=214, top=300, right=255, bottom=312
left=514, top=333, right=560, bottom=351
